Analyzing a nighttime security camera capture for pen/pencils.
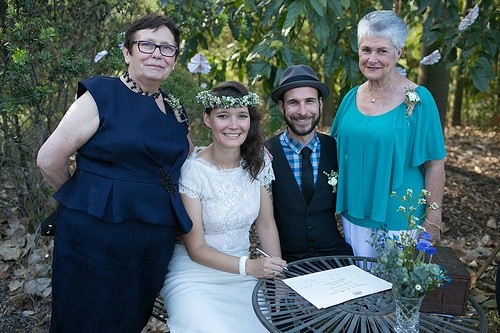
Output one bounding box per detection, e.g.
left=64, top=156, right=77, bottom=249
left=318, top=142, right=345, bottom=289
left=256, top=248, right=289, bottom=271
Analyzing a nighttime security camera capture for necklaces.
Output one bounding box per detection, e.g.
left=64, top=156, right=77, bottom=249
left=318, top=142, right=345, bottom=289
left=367, top=78, right=397, bottom=103
left=123, top=71, right=161, bottom=99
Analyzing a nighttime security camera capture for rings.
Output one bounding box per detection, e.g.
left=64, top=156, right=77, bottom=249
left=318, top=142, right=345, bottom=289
left=272, top=271, right=275, bottom=275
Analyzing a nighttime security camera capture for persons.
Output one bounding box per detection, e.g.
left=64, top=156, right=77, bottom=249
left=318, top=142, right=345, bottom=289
left=330, top=10, right=446, bottom=271
left=260, top=65, right=357, bottom=333
left=159, top=80, right=290, bottom=333
left=37, top=14, right=193, bottom=333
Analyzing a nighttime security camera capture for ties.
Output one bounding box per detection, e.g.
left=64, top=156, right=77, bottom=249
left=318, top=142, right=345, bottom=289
left=299, top=147, right=314, bottom=205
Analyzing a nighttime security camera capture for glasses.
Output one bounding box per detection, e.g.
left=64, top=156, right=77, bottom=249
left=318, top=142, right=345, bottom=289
left=132, top=40, right=178, bottom=57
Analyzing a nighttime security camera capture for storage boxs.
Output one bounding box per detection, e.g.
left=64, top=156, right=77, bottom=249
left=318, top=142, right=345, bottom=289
left=416, top=246, right=471, bottom=315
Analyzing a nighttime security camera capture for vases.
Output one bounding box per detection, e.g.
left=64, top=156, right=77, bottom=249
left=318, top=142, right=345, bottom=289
left=391, top=291, right=426, bottom=333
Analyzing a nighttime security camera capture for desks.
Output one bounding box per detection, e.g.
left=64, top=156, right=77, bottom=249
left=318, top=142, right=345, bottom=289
left=252, top=255, right=488, bottom=333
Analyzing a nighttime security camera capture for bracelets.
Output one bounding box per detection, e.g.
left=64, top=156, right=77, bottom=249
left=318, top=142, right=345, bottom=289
left=238, top=256, right=249, bottom=276
left=425, top=219, right=442, bottom=229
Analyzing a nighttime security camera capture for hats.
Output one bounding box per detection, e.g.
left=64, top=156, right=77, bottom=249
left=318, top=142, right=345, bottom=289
left=270, top=64, right=329, bottom=105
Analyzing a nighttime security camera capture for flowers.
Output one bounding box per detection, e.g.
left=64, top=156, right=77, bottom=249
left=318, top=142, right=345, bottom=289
left=405, top=84, right=421, bottom=117
left=196, top=90, right=259, bottom=110
left=163, top=93, right=181, bottom=110
left=365, top=187, right=453, bottom=298
left=323, top=170, right=339, bottom=194
